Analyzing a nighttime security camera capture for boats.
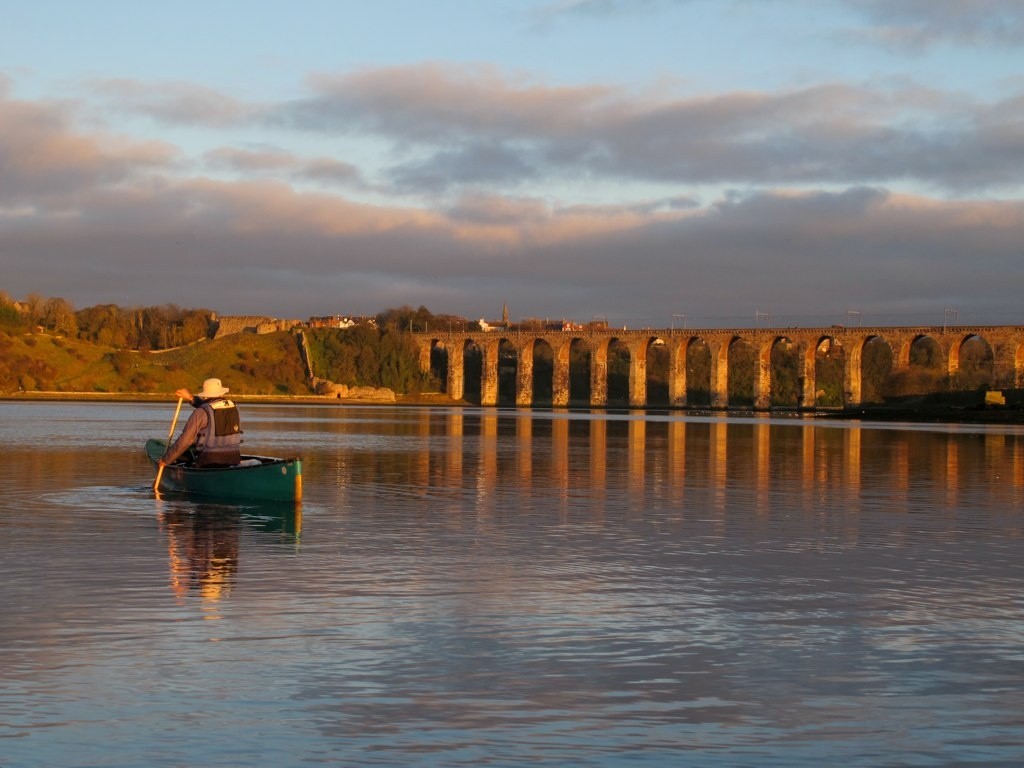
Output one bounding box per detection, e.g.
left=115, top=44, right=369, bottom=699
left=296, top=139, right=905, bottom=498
left=146, top=437, right=304, bottom=503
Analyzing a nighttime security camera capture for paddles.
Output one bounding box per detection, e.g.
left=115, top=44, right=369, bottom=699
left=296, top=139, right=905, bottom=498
left=152, top=397, right=183, bottom=491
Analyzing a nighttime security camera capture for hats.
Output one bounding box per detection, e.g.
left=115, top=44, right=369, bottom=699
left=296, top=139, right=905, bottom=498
left=193, top=378, right=230, bottom=397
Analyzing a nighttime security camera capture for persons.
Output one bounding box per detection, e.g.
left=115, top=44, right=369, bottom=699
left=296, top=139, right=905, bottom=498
left=158, top=378, right=244, bottom=467
